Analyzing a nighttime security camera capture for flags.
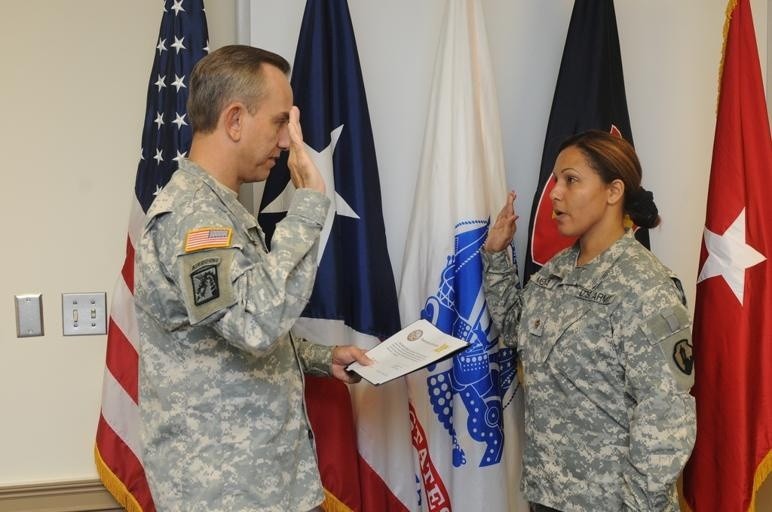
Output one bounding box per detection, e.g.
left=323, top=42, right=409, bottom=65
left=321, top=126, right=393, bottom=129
left=678, top=0, right=772, bottom=512
left=523, top=0, right=651, bottom=285
left=94, top=1, right=212, bottom=512
left=251, top=0, right=417, bottom=512
left=396, top=0, right=530, bottom=512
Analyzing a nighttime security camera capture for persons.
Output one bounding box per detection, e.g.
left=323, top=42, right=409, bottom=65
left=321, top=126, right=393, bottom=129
left=133, top=45, right=373, bottom=512
left=478, top=128, right=698, bottom=512
